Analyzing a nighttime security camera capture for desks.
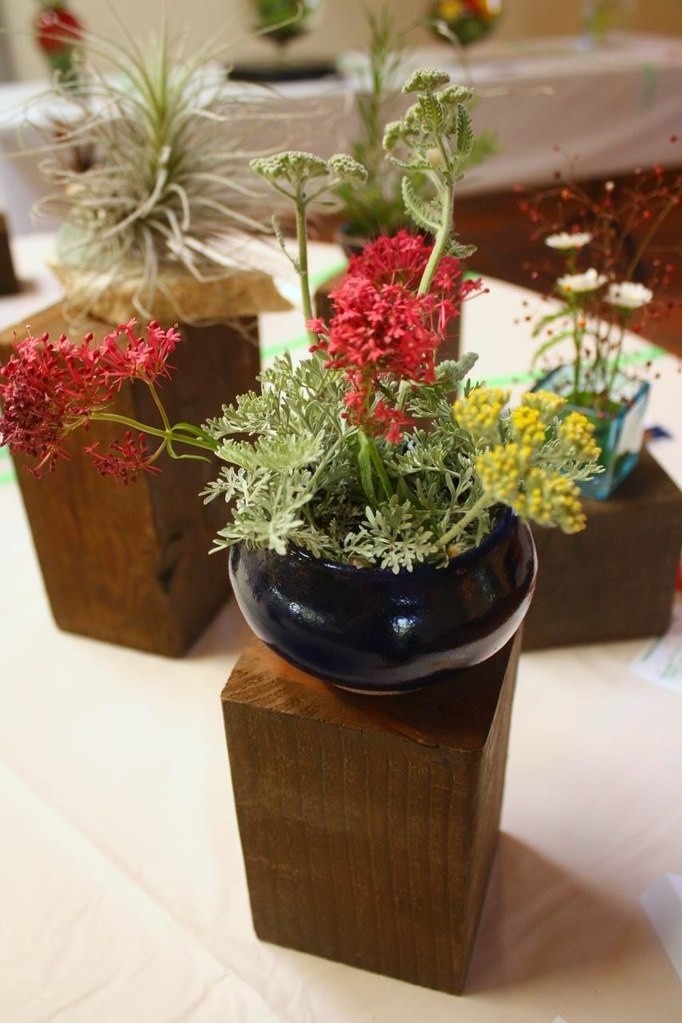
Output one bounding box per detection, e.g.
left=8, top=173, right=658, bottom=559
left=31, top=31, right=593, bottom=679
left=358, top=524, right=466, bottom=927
left=0, top=31, right=682, bottom=277
left=0, top=239, right=682, bottom=1023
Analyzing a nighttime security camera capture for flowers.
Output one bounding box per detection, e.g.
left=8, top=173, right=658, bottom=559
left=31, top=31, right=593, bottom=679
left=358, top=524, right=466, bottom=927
left=0, top=133, right=682, bottom=573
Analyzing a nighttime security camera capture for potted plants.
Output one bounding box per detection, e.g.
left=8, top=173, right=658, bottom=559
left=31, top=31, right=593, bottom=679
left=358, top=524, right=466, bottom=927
left=0, top=0, right=294, bottom=327
left=330, top=0, right=481, bottom=260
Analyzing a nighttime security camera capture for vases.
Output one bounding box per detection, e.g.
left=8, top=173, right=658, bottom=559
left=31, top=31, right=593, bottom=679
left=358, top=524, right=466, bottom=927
left=228, top=478, right=539, bottom=695
left=530, top=365, right=650, bottom=500
